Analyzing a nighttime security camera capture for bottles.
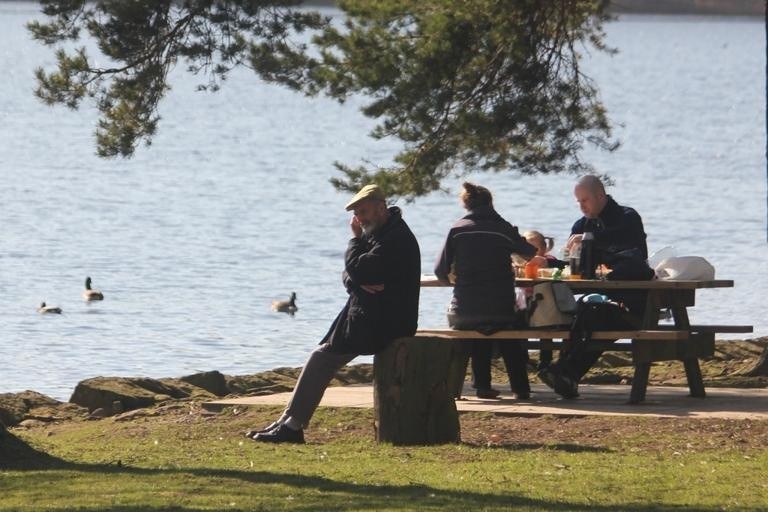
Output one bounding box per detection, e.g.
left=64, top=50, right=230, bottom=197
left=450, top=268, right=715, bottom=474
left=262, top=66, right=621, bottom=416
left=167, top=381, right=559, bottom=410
left=579, top=231, right=596, bottom=280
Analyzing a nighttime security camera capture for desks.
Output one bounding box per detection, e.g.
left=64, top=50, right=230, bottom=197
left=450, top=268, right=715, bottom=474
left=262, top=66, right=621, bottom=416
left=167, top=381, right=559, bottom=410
left=420, top=280, right=735, bottom=404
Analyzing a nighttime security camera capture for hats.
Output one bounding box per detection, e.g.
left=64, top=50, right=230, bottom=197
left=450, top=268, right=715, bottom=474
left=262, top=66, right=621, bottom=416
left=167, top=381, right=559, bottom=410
left=344, top=185, right=386, bottom=212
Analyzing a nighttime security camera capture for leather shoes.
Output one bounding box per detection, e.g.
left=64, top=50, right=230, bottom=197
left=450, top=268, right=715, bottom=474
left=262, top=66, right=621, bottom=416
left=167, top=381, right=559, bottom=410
left=246, top=421, right=304, bottom=443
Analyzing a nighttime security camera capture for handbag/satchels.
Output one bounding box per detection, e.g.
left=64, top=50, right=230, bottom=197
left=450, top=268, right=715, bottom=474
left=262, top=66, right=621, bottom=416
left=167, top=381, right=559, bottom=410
left=527, top=278, right=632, bottom=329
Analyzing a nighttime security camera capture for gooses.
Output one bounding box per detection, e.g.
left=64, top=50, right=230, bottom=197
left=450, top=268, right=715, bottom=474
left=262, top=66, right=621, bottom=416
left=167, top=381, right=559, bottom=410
left=37, top=302, right=62, bottom=314
left=270, top=292, right=299, bottom=315
left=84, top=276, right=104, bottom=301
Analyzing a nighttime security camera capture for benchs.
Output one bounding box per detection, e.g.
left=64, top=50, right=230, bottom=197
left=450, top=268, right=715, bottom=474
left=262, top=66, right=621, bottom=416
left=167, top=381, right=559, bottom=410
left=634, top=325, right=754, bottom=398
left=414, top=329, right=688, bottom=405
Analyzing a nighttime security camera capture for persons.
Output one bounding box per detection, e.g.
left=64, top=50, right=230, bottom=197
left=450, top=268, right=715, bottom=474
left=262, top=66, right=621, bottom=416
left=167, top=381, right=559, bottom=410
left=512, top=231, right=557, bottom=372
left=538, top=175, right=657, bottom=399
left=434, top=182, right=539, bottom=400
left=243, top=181, right=421, bottom=443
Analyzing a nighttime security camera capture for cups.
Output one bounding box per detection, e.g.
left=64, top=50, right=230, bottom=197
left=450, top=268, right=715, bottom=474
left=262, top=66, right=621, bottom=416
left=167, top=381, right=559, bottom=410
left=568, top=256, right=580, bottom=275
left=525, top=262, right=538, bottom=279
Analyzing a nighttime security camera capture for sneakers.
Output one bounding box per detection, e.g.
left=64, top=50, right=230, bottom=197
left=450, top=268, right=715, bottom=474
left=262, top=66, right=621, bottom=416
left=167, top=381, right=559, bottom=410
left=476, top=386, right=531, bottom=398
left=536, top=368, right=578, bottom=396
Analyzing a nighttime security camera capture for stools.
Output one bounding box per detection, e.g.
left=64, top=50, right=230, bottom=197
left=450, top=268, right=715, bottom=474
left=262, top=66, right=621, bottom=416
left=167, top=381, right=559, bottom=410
left=372, top=336, right=462, bottom=446
